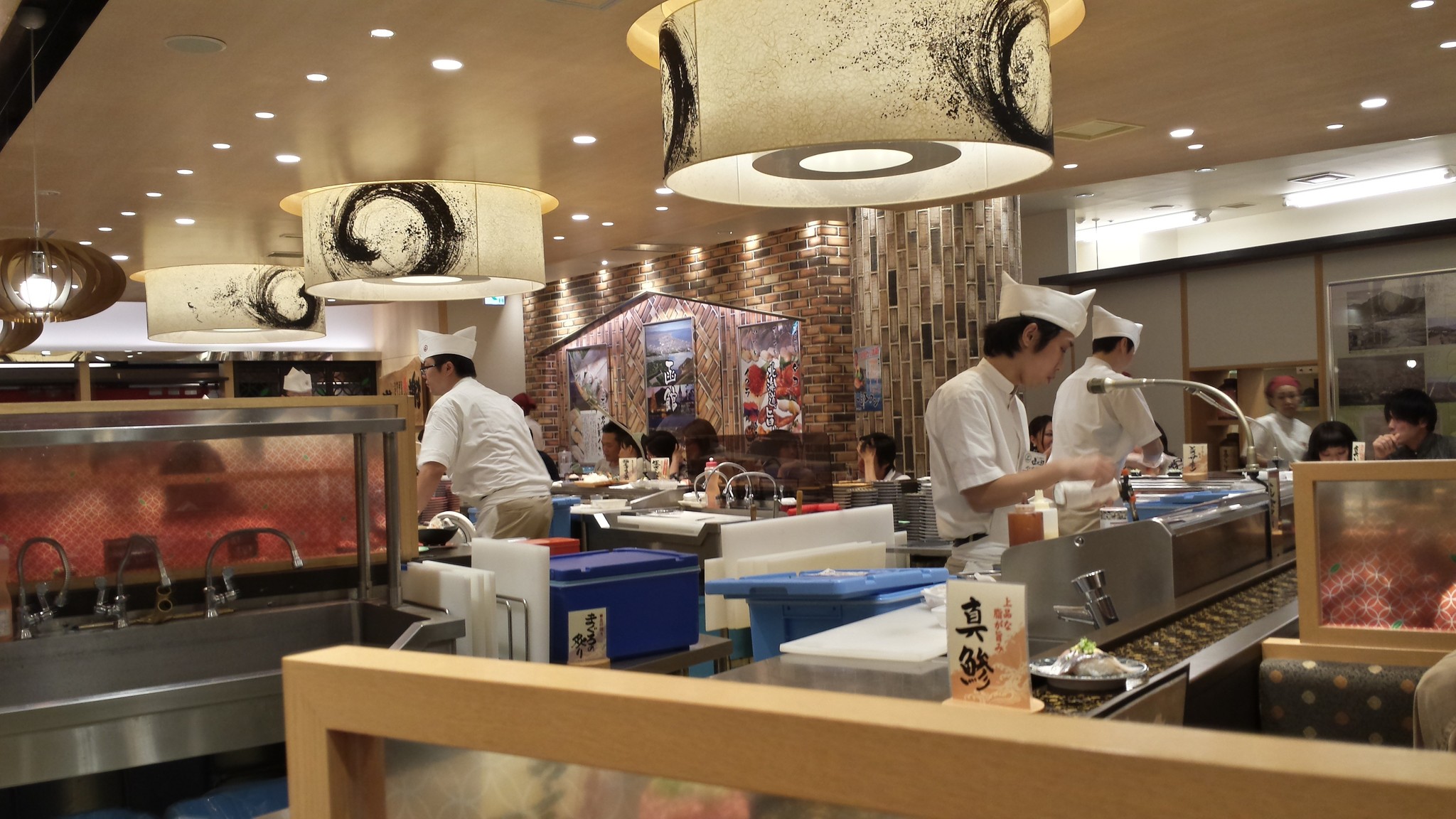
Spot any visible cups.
[590,494,603,500]
[572,495,582,505]
[571,463,583,474]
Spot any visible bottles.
[704,457,720,508]
[1215,378,1237,420]
[1007,492,1044,547]
[1031,490,1057,508]
[1219,433,1242,473]
[1034,502,1059,540]
[1122,469,1139,521]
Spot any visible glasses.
[420,362,441,375]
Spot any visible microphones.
[1179,383,1292,486]
[1087,376,1267,492]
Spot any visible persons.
[857,433,911,482]
[618,433,642,459]
[194,394,255,472]
[1028,305,1183,539]
[262,367,354,472]
[416,325,561,540]
[682,418,734,484]
[1372,388,1456,460]
[645,430,688,483]
[1240,375,1313,471]
[1302,421,1366,461]
[924,271,1117,576]
[756,429,822,487]
[37,440,284,580]
[593,421,628,481]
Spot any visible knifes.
[621,512,678,517]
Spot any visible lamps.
[1075,209,1212,242]
[0,6,127,355]
[625,1,1087,209]
[130,264,327,344]
[1279,165,1456,209]
[280,179,560,302]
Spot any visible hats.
[1266,375,1300,408]
[417,326,477,364]
[512,392,538,410]
[998,270,1097,337]
[1092,304,1143,355]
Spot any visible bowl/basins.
[590,498,627,509]
[418,525,460,546]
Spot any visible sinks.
[1,590,467,793]
[621,505,719,521]
[695,514,766,525]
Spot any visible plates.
[833,480,946,541]
[1029,655,1148,690]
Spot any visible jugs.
[558,450,572,478]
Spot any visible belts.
[953,533,988,547]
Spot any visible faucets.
[93,534,172,629]
[203,528,304,618]
[722,470,784,518]
[701,462,754,508]
[693,469,736,508]
[15,537,72,639]
[1053,568,1121,632]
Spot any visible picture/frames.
[642,317,698,463]
[566,343,612,465]
[737,319,805,460]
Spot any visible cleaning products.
[0,544,14,642]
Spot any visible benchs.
[1259,658,1431,747]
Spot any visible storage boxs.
[468,497,581,538]
[1123,491,1248,522]
[704,567,956,662]
[549,547,701,665]
